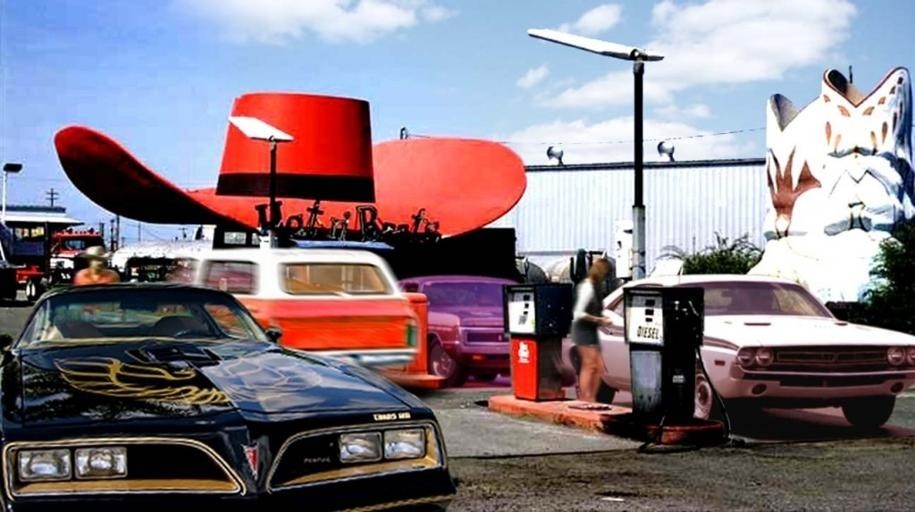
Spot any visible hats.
[54,91,528,237]
[76,246,114,259]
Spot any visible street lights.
[2,162,24,227]
[527,26,667,278]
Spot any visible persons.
[72,246,120,285]
[570,258,613,404]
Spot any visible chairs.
[43,320,103,343]
[152,316,214,341]
